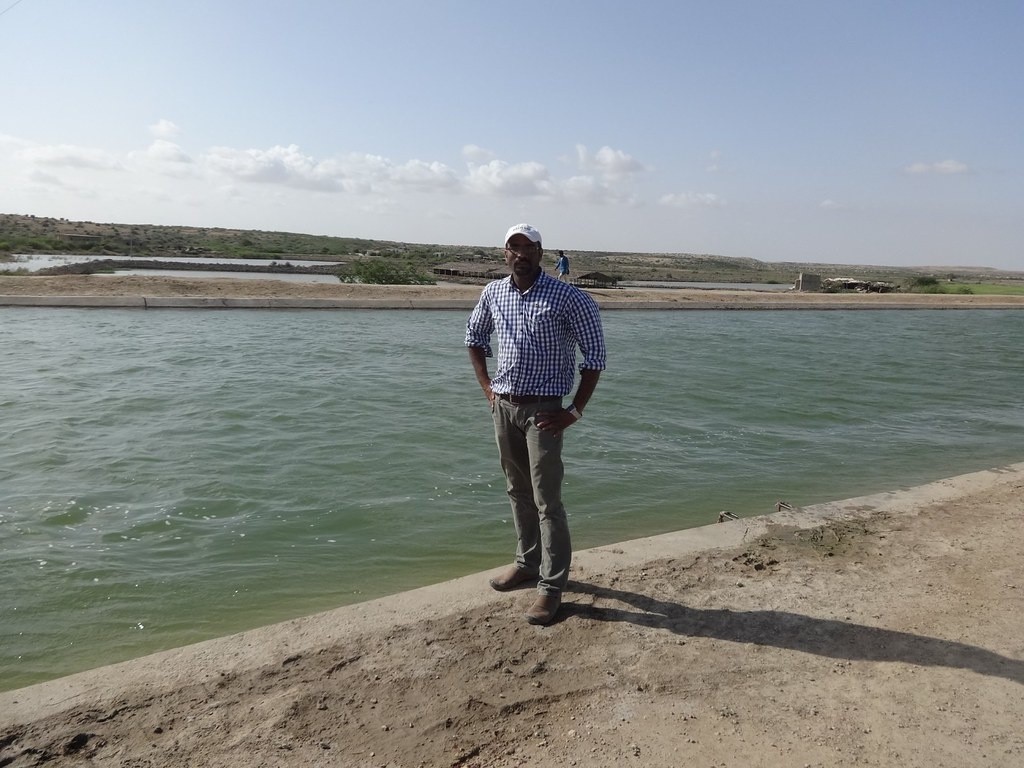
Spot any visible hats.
[504,223,541,248]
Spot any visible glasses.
[506,244,540,254]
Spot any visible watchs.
[567,404,583,420]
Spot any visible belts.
[494,393,559,404]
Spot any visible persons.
[464,223,608,626]
[555,250,569,284]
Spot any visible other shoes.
[524,595,560,626]
[489,566,533,591]
